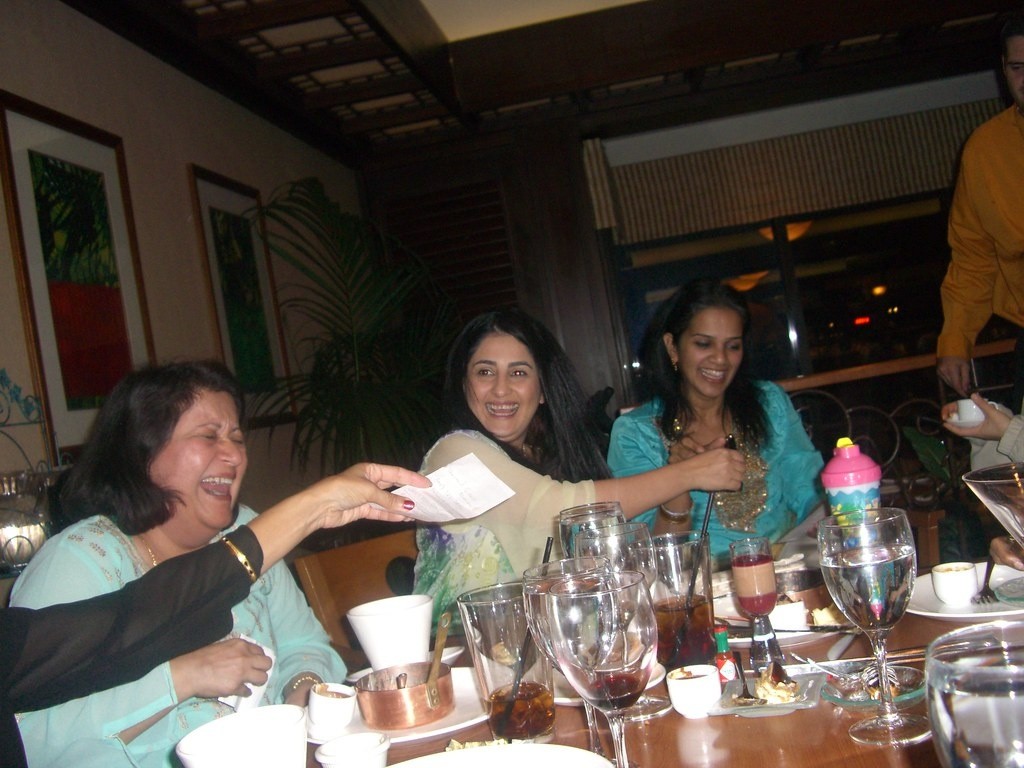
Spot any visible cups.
[932,562,978,604]
[962,460,1024,550]
[308,682,357,729]
[666,664,722,718]
[175,703,306,768]
[626,529,719,685]
[313,732,391,768]
[924,619,1024,768]
[957,398,998,422]
[347,593,434,672]
[458,579,557,744]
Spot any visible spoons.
[732,651,768,706]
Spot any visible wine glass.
[521,500,673,767]
[814,506,932,749]
[728,536,787,670]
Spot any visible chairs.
[294,528,418,655]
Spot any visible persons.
[605,280,831,574]
[940,393,1024,474]
[1,460,434,716]
[933,23,1024,404]
[0,356,348,768]
[410,311,747,638]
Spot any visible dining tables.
[306,585,979,768]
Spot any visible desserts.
[755,662,799,704]
[810,601,851,625]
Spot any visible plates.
[525,660,667,706]
[906,560,1024,622]
[706,671,826,718]
[712,593,842,651]
[305,665,492,746]
[947,416,983,427]
[380,743,613,767]
[346,645,465,682]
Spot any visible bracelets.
[658,500,695,526]
[136,530,157,569]
[222,536,258,585]
[292,675,321,689]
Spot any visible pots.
[354,612,456,730]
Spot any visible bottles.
[820,437,884,553]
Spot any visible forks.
[806,654,932,678]
[971,556,999,605]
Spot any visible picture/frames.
[0,89,159,472]
[187,162,297,429]
